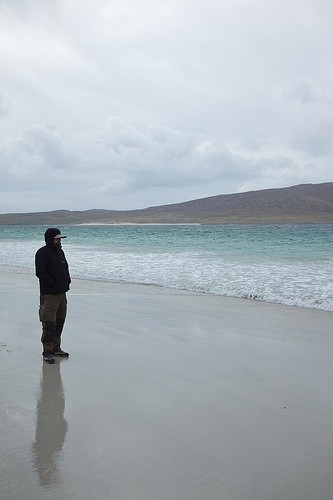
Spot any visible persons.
[29,362,69,490]
[33,228,72,364]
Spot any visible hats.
[53,233,69,240]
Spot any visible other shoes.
[42,353,56,365]
[53,349,69,357]
